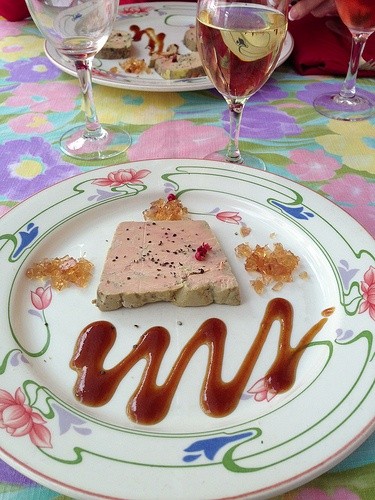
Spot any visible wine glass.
[313,0,375,119]
[198,1,290,172]
[26,0,133,161]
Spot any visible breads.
[91,30,134,60]
[148,50,214,78]
[96,220,242,309]
[183,25,200,51]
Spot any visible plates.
[42,2,294,92]
[1,159,375,499]
